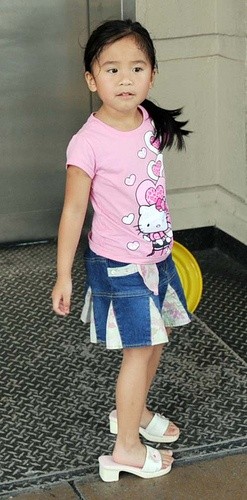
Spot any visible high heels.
[97,444,172,482]
[109,407,180,442]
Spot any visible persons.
[51,18,192,484]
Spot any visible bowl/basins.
[164,239,203,317]
